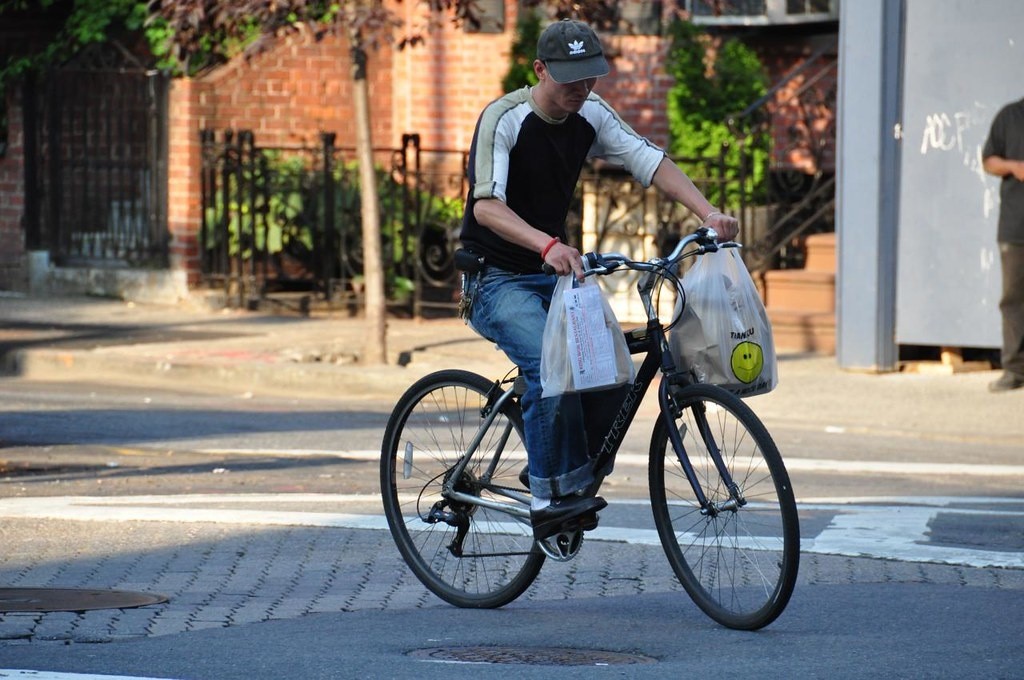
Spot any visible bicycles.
[379,225,800,631]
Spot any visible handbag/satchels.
[672,240,777,398]
[539,257,635,399]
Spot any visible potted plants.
[662,8,781,278]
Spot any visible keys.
[458,291,471,325]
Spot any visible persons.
[982,94,1024,391]
[458,17,739,562]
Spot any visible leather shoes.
[530,491,606,540]
[520,465,532,486]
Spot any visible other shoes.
[989,370,1024,392]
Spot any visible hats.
[536,18,609,85]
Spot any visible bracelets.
[703,212,721,223]
[541,237,560,260]
